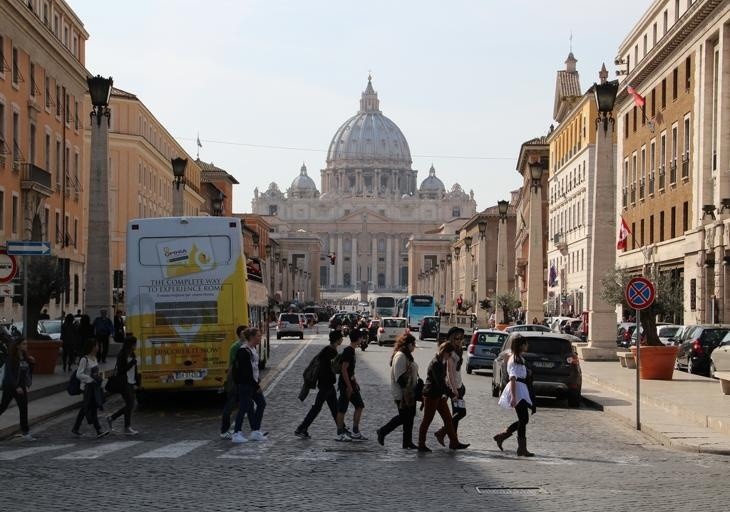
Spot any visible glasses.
[455,336,464,340]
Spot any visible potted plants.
[490,289,518,331]
[598,261,689,380]
[10,258,69,375]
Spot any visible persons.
[294,330,352,439]
[341,315,370,331]
[232,328,267,444]
[493,333,536,457]
[334,329,368,441]
[418,341,461,452]
[220,326,255,439]
[0,309,140,442]
[434,326,471,450]
[376,334,424,449]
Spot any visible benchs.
[712,371,730,395]
[616,352,636,370]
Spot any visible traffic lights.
[330,255,335,264]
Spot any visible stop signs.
[457,297,463,304]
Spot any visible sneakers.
[334,426,368,442]
[294,431,311,439]
[105,415,112,433]
[96,431,110,439]
[24,434,38,442]
[123,427,139,436]
[71,430,85,438]
[232,431,270,443]
[219,432,233,440]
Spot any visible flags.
[617,217,630,250]
[549,264,556,286]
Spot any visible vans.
[462,328,510,375]
[417,316,441,341]
[376,317,410,346]
[276,313,304,340]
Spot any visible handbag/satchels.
[412,379,425,411]
[105,376,123,393]
[67,369,81,395]
[0,363,6,390]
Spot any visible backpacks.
[330,349,354,374]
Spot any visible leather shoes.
[403,442,419,449]
[453,442,470,449]
[419,446,432,453]
[377,429,384,445]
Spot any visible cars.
[709,331,730,378]
[300,305,379,351]
[489,330,582,408]
[544,315,589,342]
[615,321,730,374]
[9,319,75,352]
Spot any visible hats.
[83,338,96,353]
[350,329,365,341]
[446,326,464,341]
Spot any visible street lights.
[264,244,273,298]
[274,251,312,303]
[495,199,510,329]
[525,160,547,326]
[252,231,261,257]
[210,188,223,217]
[172,157,187,217]
[576,80,631,361]
[417,219,488,328]
[85,74,123,358]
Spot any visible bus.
[123,215,280,412]
[369,296,397,321]
[396,296,405,317]
[401,294,436,330]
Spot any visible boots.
[435,426,448,447]
[493,431,513,451]
[517,437,534,457]
